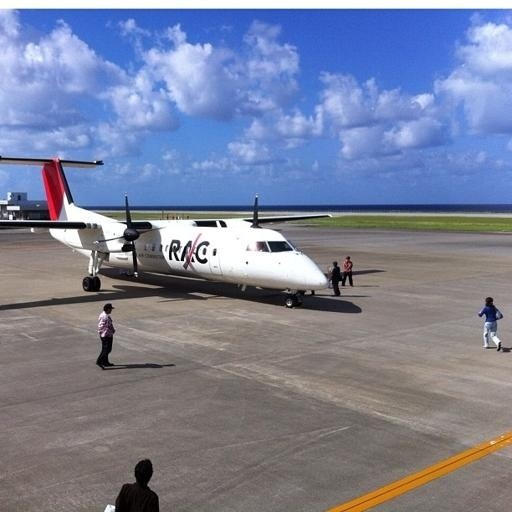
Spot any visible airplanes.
[0,156,330,307]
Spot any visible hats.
[104,304,115,311]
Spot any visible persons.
[477,296,504,352]
[328,261,342,297]
[114,458,159,512]
[342,255,354,287]
[94,303,116,370]
